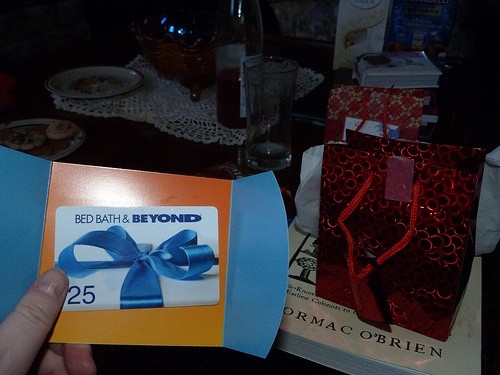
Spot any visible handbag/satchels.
[314,137,488,342]
[289,140,341,234]
[319,84,422,145]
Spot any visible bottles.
[216,0,263,127]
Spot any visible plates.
[46,65,143,101]
[2,118,83,160]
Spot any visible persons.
[1,268,97,375]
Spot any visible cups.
[241,55,299,171]
